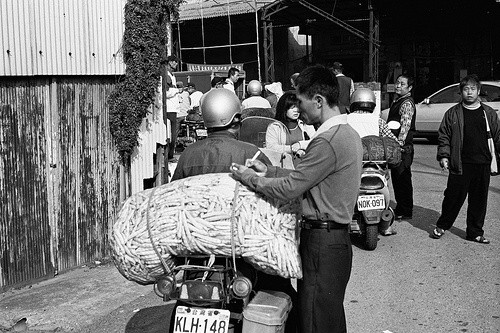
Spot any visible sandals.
[466,236,490,243]
[432,226,446,237]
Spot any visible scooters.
[174,116,208,154]
[153,254,294,333]
[351,120,401,251]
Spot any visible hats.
[187,83,194,88]
[330,62,344,71]
[264,82,282,95]
[212,77,228,85]
[167,55,181,61]
[176,82,185,88]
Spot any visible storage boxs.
[242,289,292,333]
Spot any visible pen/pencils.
[246,150,261,168]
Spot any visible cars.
[381,80,500,144]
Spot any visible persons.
[230,64,363,333]
[432,75,500,244]
[170,88,272,183]
[165,56,180,162]
[387,74,416,220]
[266,91,311,160]
[175,67,301,130]
[330,62,355,114]
[345,87,402,235]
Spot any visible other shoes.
[393,208,412,220]
[384,227,398,235]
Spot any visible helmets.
[200,88,242,127]
[350,87,376,104]
[247,80,263,95]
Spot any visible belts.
[298,219,349,229]
